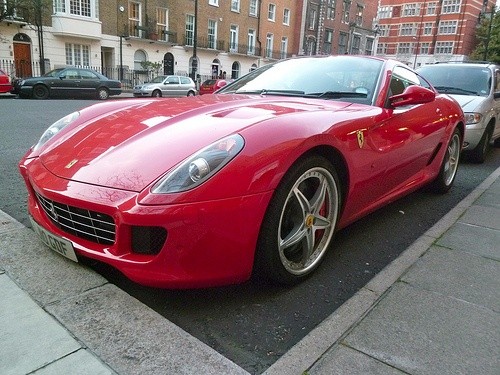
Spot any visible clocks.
[120,6,125,12]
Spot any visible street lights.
[119,35,130,82]
[480,0,500,63]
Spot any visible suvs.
[418,61,500,165]
[135,76,198,97]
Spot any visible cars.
[0,70,13,94]
[13,68,123,100]
[200,79,227,95]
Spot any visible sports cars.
[17,54,467,290]
